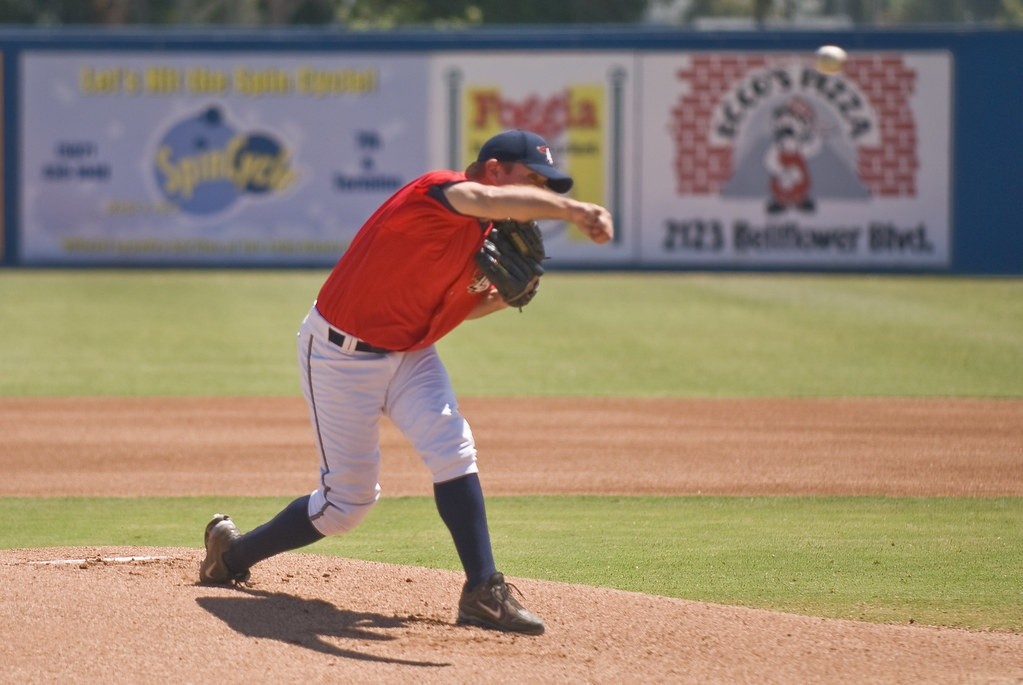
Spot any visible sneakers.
[456,571,545,636]
[199,515,252,586]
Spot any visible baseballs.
[814,44,849,76]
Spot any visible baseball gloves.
[474,217,552,314]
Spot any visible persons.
[200,129,614,636]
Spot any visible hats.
[477,128,573,194]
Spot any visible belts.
[329,329,389,353]
